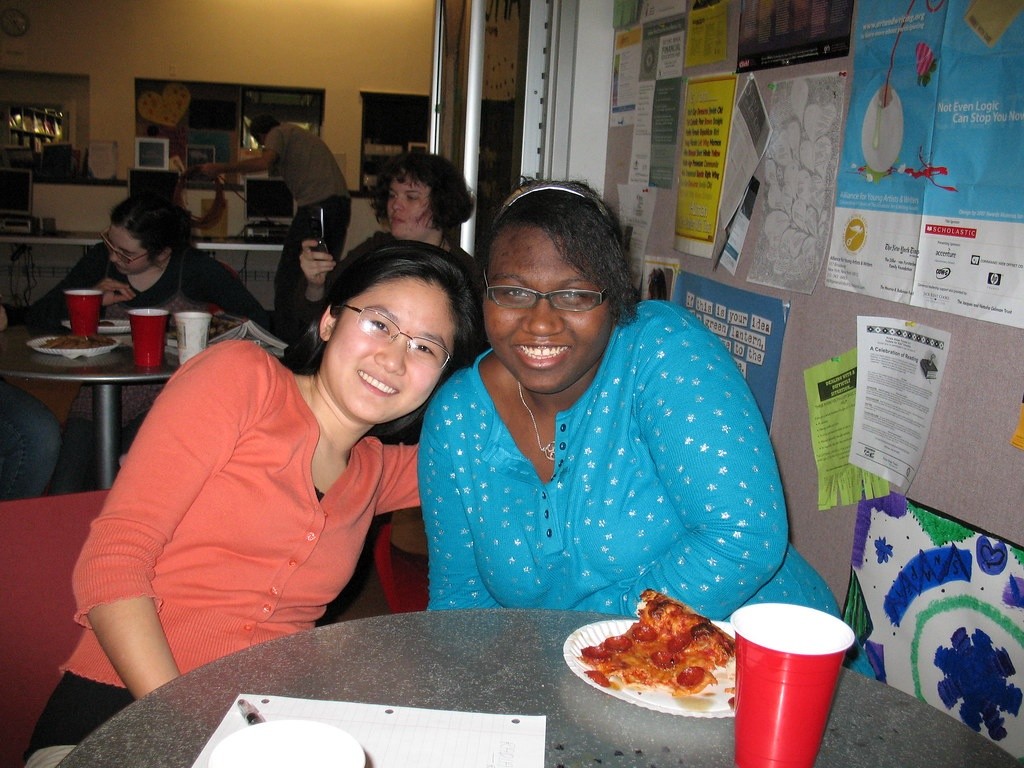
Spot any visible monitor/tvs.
[246,177,295,224]
[0,167,33,215]
[127,167,176,206]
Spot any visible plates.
[211,721,366,768]
[564,618,751,721]
[61,318,133,334]
[26,335,121,358]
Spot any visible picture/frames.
[134,138,170,171]
[186,144,215,169]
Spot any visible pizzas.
[580,587,737,698]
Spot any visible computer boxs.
[0,215,38,236]
[244,222,289,244]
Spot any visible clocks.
[0,6,27,35]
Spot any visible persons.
[275,152,491,527]
[24,240,489,768]
[250,114,353,338]
[0,193,270,497]
[418,180,875,683]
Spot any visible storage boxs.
[31,180,129,233]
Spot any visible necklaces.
[517,381,554,461]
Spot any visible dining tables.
[1,315,275,490]
[53,608,1024,768]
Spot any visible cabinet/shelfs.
[0,102,65,153]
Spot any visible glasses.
[345,304,452,370]
[481,269,606,312]
[100,227,151,265]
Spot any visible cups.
[174,311,213,367]
[128,308,170,367]
[732,601,858,768]
[64,289,103,338]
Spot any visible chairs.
[0,490,109,768]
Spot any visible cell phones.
[308,205,330,255]
